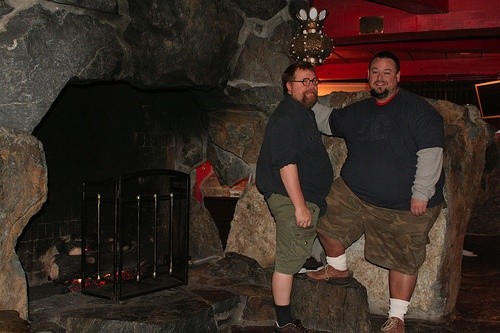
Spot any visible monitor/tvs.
[475,80,500,119]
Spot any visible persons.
[306,50,448,333]
[255,61,334,333]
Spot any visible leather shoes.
[380,316,405,333]
[306,264,351,284]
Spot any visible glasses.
[288,77,320,86]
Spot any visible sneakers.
[273,319,313,333]
[298,256,325,274]
